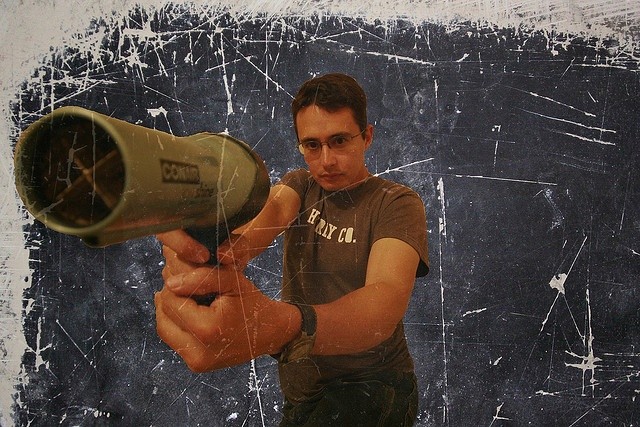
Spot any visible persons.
[154,73,429,426]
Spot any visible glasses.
[298,129,365,156]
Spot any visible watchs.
[269,300,316,365]
